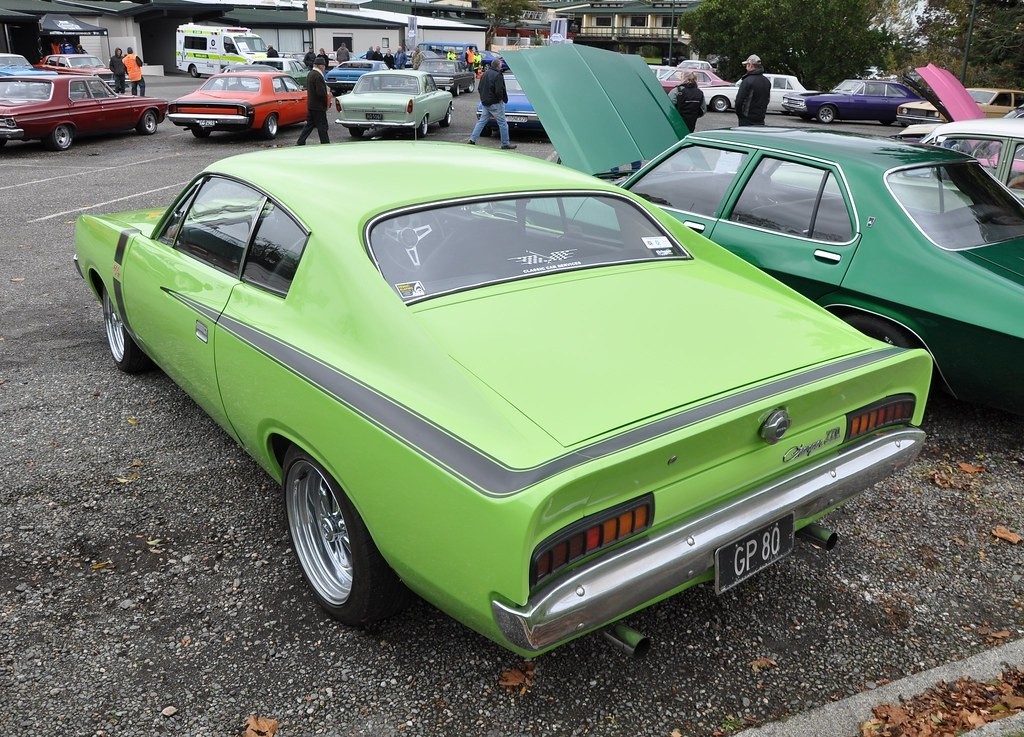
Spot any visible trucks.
[176,22,269,78]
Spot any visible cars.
[700,73,819,114]
[355,41,510,73]
[769,63,1024,214]
[212,64,298,91]
[324,60,391,96]
[334,70,454,138]
[0,53,59,93]
[253,51,358,82]
[32,54,115,92]
[165,71,334,140]
[650,60,734,94]
[465,41,1024,414]
[477,75,547,137]
[408,59,475,97]
[0,76,168,151]
[74,141,934,661]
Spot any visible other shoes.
[501,145,517,149]
[467,140,475,145]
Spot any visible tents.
[39,13,111,60]
[0,8,37,54]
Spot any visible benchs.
[747,197,847,238]
[71,92,85,99]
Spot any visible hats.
[313,58,326,64]
[742,54,761,65]
[309,46,312,49]
[127,47,132,52]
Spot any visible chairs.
[228,83,246,90]
[859,87,870,94]
[416,216,524,277]
[872,87,881,94]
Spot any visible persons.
[316,49,329,76]
[735,54,771,127]
[304,47,316,71]
[447,48,457,67]
[384,49,394,70]
[467,59,517,149]
[110,48,128,94]
[373,46,384,61]
[366,46,374,60]
[51,38,88,55]
[465,46,474,73]
[337,43,350,64]
[296,57,330,145]
[474,50,482,74]
[411,46,422,71]
[668,69,707,132]
[267,45,279,67]
[394,46,407,69]
[123,47,145,96]
